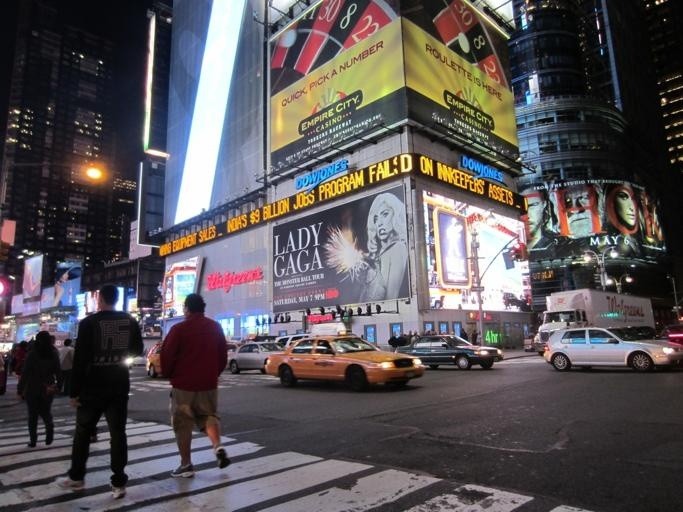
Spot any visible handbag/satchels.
[40,380,59,397]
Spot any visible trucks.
[532,290,659,355]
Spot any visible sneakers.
[215,447,232,470]
[54,475,85,491]
[111,482,126,499]
[170,464,195,478]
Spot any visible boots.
[28,421,37,447]
[45,423,54,445]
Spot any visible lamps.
[252,300,383,327]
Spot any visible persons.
[161,294,231,479]
[522,178,667,264]
[57,285,142,500]
[356,194,407,304]
[1,331,81,449]
[390,327,478,350]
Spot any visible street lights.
[0,159,104,186]
[580,244,615,291]
[666,275,680,322]
[466,227,484,347]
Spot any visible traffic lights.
[514,244,525,261]
